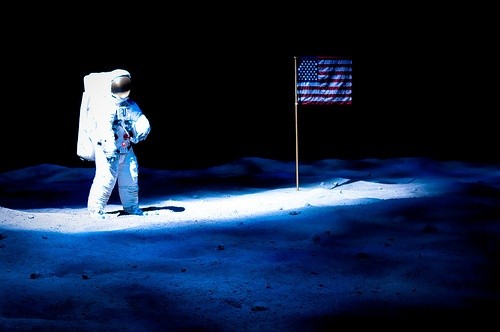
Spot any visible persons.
[87,67,152,217]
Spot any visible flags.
[296,57,353,105]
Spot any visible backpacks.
[76,72,108,162]
